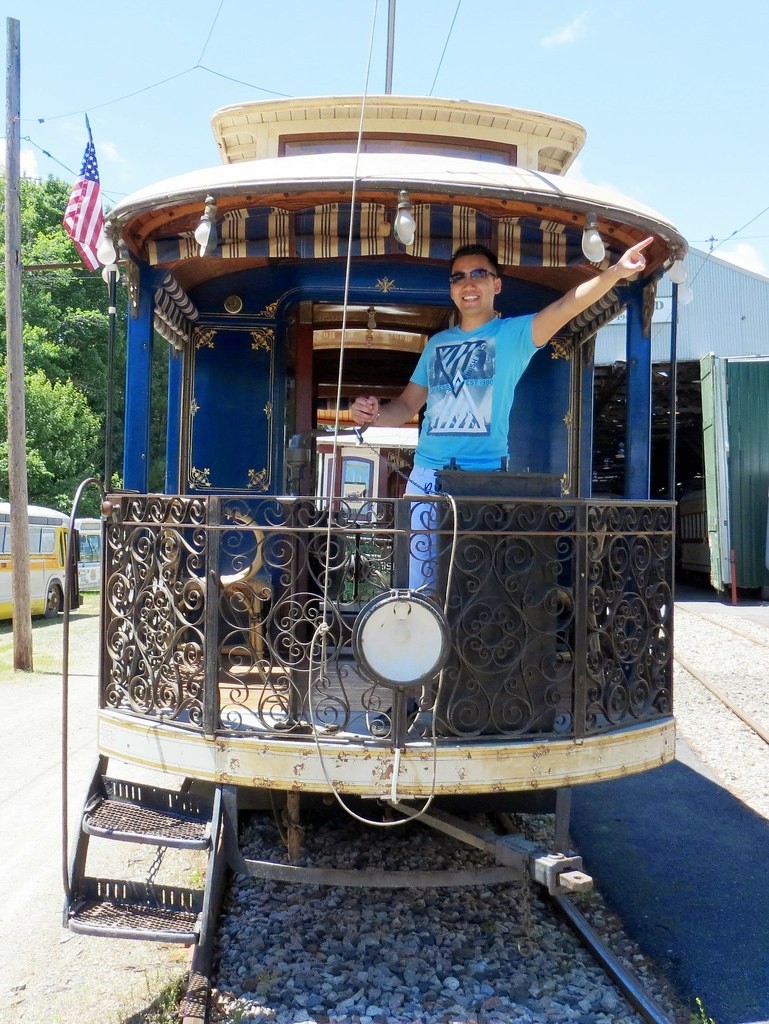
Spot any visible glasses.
[449,268,496,285]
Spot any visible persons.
[347,238,655,735]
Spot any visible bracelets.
[371,407,381,425]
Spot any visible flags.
[64,138,111,273]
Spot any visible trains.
[57,93,688,943]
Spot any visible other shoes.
[370,699,419,734]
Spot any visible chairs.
[111,486,273,684]
[412,452,609,722]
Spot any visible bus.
[74,518,101,592]
[0,502,80,625]
